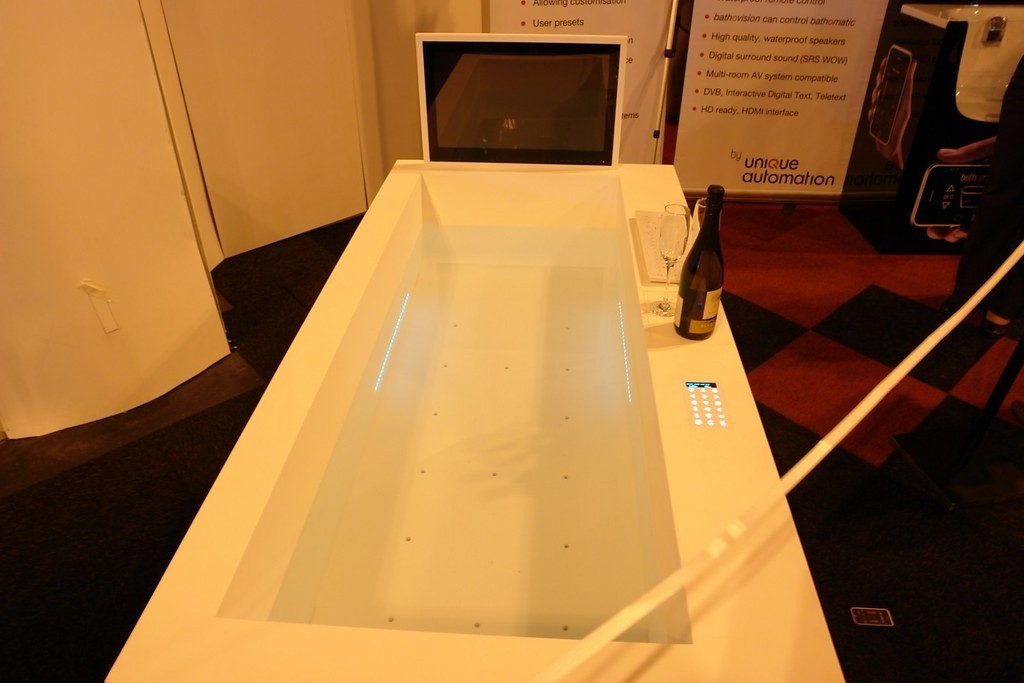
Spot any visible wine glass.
[688,197,722,252]
[651,204,690,317]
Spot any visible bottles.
[673,184,726,341]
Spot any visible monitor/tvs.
[414,31,628,173]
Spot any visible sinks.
[97,157,851,683]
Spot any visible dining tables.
[104,155,846,683]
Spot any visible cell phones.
[868,44,912,147]
[910,162,990,228]
